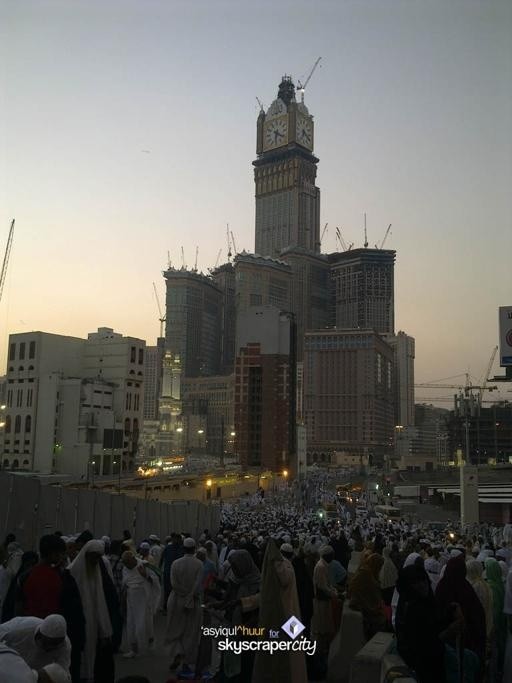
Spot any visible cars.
[355,505,368,516]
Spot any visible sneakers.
[121,649,137,658]
[169,654,193,672]
[145,637,158,650]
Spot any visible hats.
[38,612,68,639]
[41,661,70,682]
[0,508,512,575]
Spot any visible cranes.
[412,345,508,405]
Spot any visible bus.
[375,506,400,522]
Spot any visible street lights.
[395,425,404,456]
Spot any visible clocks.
[292,109,314,151]
[262,113,288,152]
